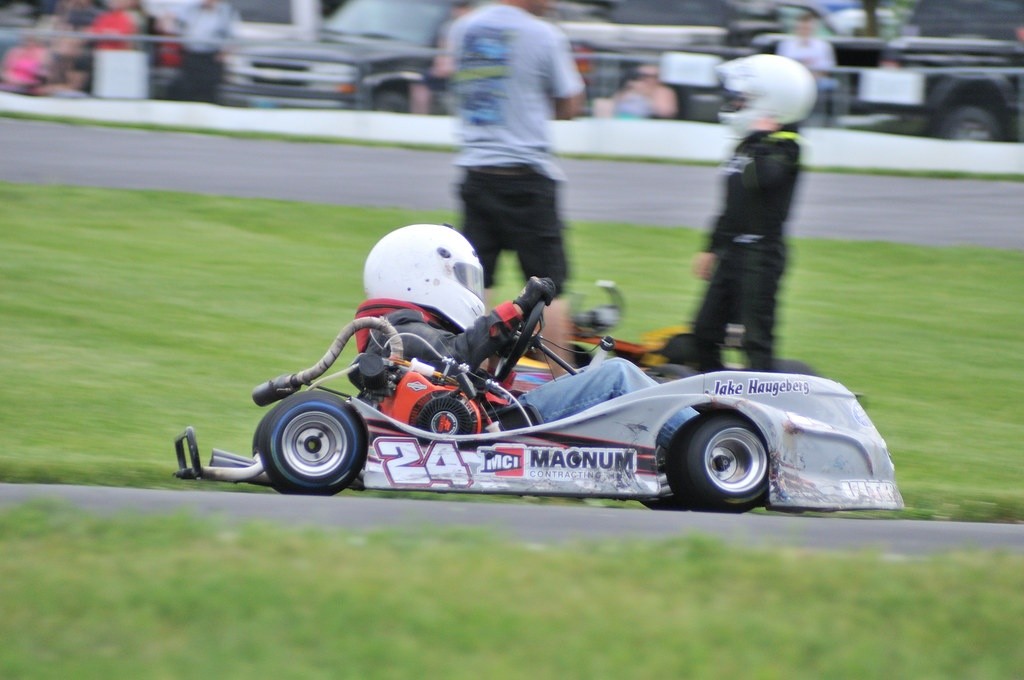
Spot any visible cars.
[2,0,852,144]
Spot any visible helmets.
[714,54,818,139]
[363,223,485,330]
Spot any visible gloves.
[513,276,556,314]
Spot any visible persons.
[689,53,817,371]
[0,0,240,102]
[778,10,836,115]
[593,63,678,119]
[447,0,585,373]
[355,224,699,448]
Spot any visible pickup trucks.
[658,0,1024,145]
